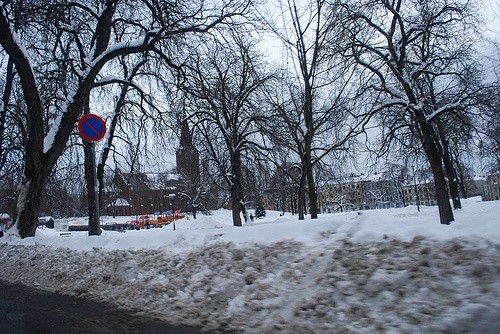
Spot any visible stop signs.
[78,113,106,142]
